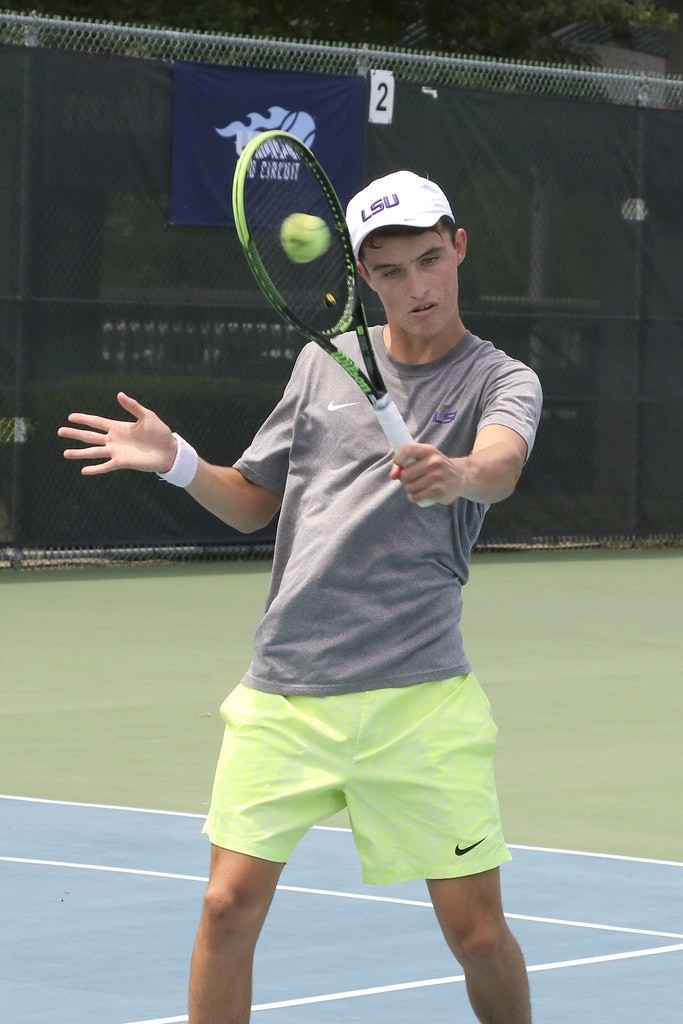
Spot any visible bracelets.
[156,432,198,488]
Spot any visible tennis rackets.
[232,128,432,511]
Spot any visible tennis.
[279,213,333,264]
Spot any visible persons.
[59,167,532,1021]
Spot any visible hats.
[345,171,456,262]
